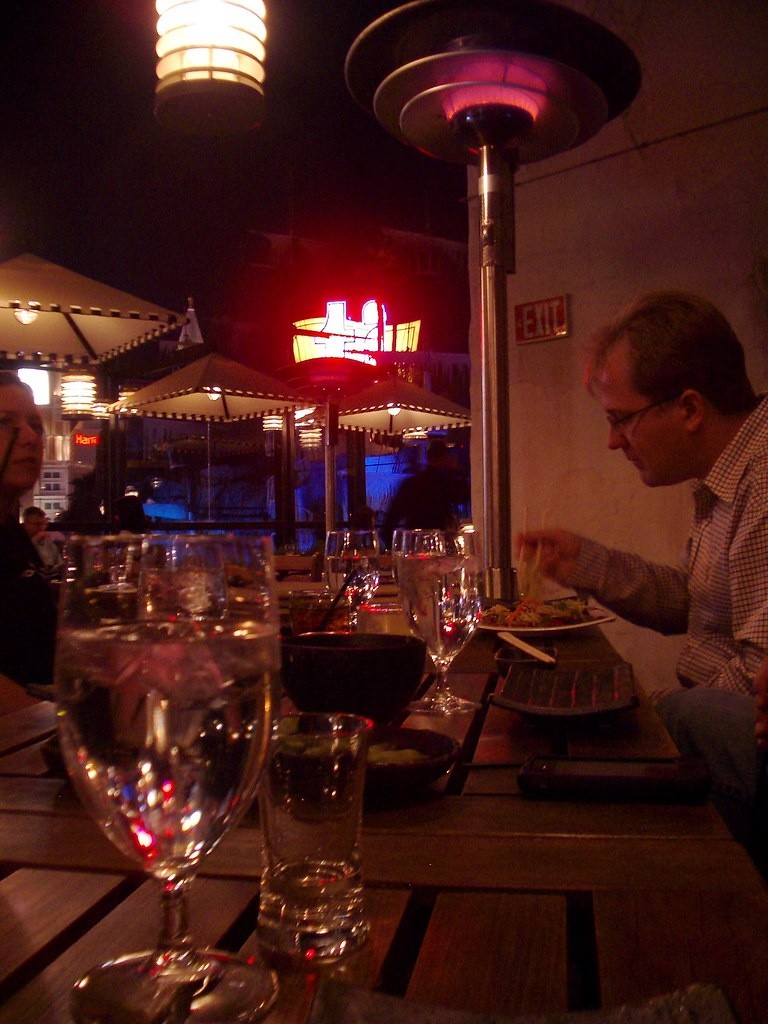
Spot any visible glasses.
[606,386,689,438]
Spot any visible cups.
[392,527,444,637]
[485,568,520,606]
[288,589,352,634]
[256,713,370,966]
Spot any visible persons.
[0,383,58,682]
[385,442,459,548]
[22,506,64,577]
[518,290,767,841]
[110,495,148,533]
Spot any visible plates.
[368,726,460,792]
[477,616,617,642]
[485,660,641,725]
[493,644,560,678]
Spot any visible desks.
[0,566,768,1024]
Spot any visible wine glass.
[397,551,484,714]
[326,531,379,630]
[55,534,279,1024]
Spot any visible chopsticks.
[516,505,549,574]
[496,631,557,664]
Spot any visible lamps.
[298,427,323,451]
[344,0,643,615]
[58,370,142,421]
[0,350,90,364]
[261,415,284,430]
[386,402,401,417]
[206,386,222,400]
[400,429,427,446]
[152,0,268,143]
[9,309,39,325]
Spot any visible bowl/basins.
[280,632,426,732]
